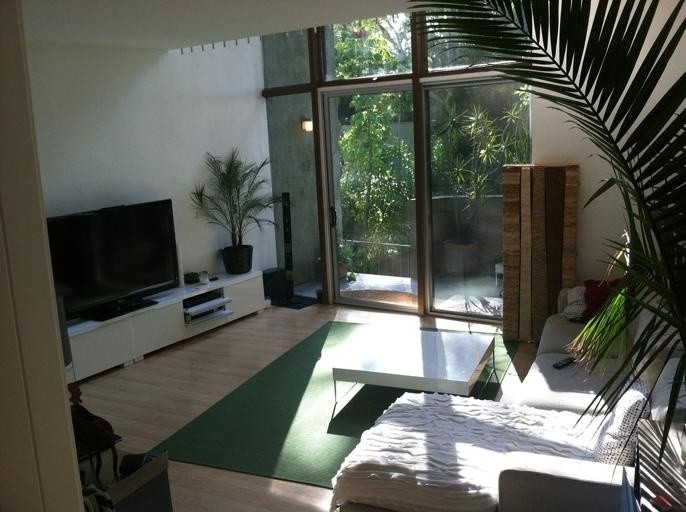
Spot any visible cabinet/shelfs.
[64,269,266,385]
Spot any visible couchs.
[328,288,686,512]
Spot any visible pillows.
[583,278,622,323]
[563,286,587,319]
[593,389,650,466]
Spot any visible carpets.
[148,321,520,490]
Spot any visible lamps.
[301,118,313,132]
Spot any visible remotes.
[553,356,577,369]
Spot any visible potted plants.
[430,85,532,277]
[190,146,295,274]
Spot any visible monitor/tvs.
[45,198,180,322]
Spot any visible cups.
[199,271,209,284]
